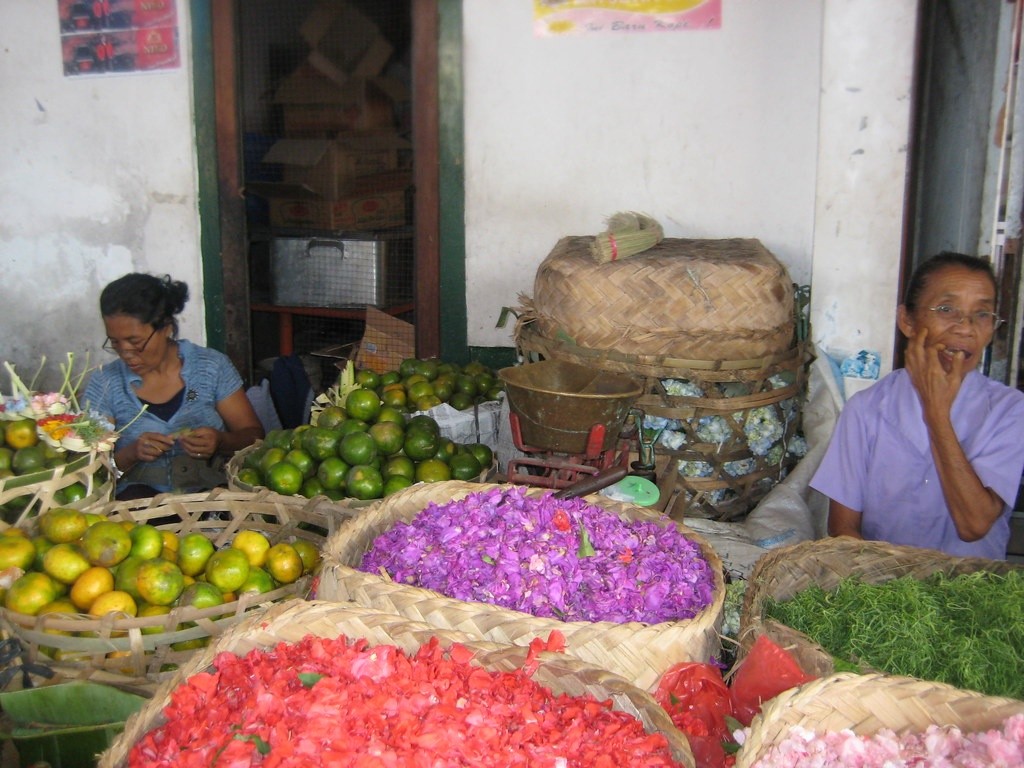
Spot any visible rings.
[198,453,200,458]
[145,440,148,446]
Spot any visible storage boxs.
[268,3,415,308]
[351,305,415,375]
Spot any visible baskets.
[513,233,813,519]
[2,418,1022,767]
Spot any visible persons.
[80,273,267,526]
[807,252,1024,561]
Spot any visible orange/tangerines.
[240,358,504,538]
[0,417,319,674]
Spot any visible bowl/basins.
[498,359,644,454]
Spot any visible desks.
[250,296,412,359]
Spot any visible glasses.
[102,320,163,355]
[907,304,1006,331]
